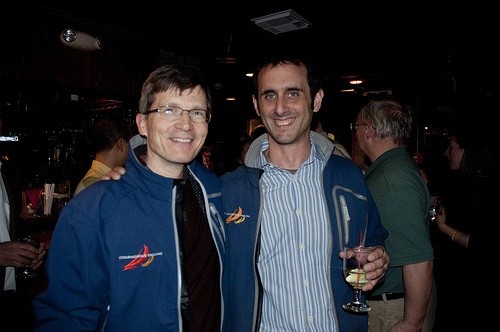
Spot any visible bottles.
[428,192,440,223]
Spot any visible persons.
[73,119,130,197]
[99,51,389,332]
[430,127,500,332]
[0,161,46,332]
[356,102,437,332]
[33,64,389,332]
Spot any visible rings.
[375,245,385,256]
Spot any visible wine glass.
[17,237,37,278]
[343,247,372,312]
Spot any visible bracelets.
[451,230,457,241]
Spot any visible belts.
[365,292,405,302]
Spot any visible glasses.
[144,106,212,123]
[350,123,377,131]
